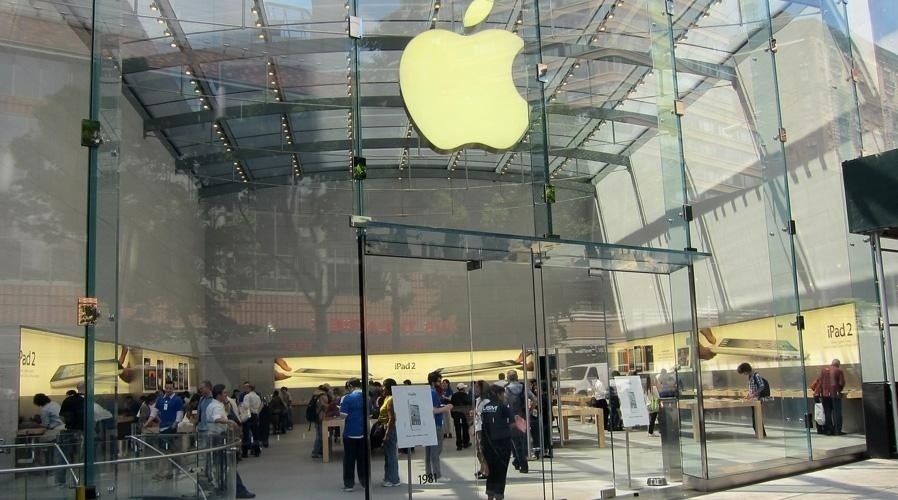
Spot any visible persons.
[192,381,255,498]
[425,371,452,482]
[481,384,516,499]
[811,359,846,435]
[311,383,343,457]
[679,348,689,366]
[441,379,454,438]
[336,376,401,492]
[77,381,113,422]
[143,381,184,481]
[403,379,411,385]
[239,381,263,457]
[137,390,202,469]
[123,395,140,423]
[35,393,64,477]
[587,368,684,437]
[268,390,287,435]
[257,391,269,448]
[451,382,472,451]
[281,387,294,430]
[55,390,85,488]
[504,369,537,474]
[468,380,490,479]
[528,378,551,459]
[737,363,767,437]
[493,373,508,387]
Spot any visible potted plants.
[142,356,189,391]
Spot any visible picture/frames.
[18,412,139,472]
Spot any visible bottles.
[457,382,468,389]
[321,383,330,390]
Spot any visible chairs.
[434,476,451,483]
[475,471,488,479]
[383,480,400,487]
[239,490,255,497]
[464,442,472,448]
[311,452,322,458]
[341,485,353,492]
[649,433,658,437]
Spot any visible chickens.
[617,344,653,373]
[433,360,523,377]
[50,359,123,388]
[284,367,383,380]
[706,336,810,360]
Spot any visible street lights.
[814,402,826,425]
[240,406,252,422]
[370,420,385,448]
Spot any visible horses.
[627,389,639,413]
[144,358,188,390]
[408,398,422,431]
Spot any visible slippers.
[647,392,659,411]
[753,372,770,397]
[306,394,325,422]
[509,388,525,416]
